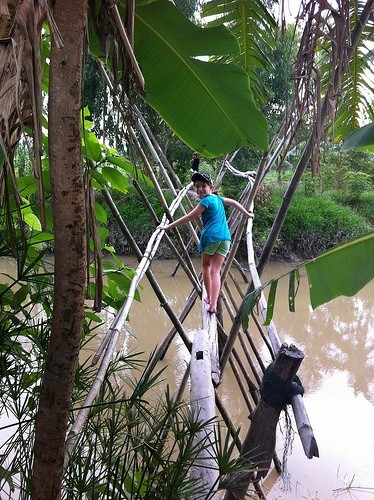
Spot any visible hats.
[190,172,213,185]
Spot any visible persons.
[156,172,254,313]
[191,154,200,178]
